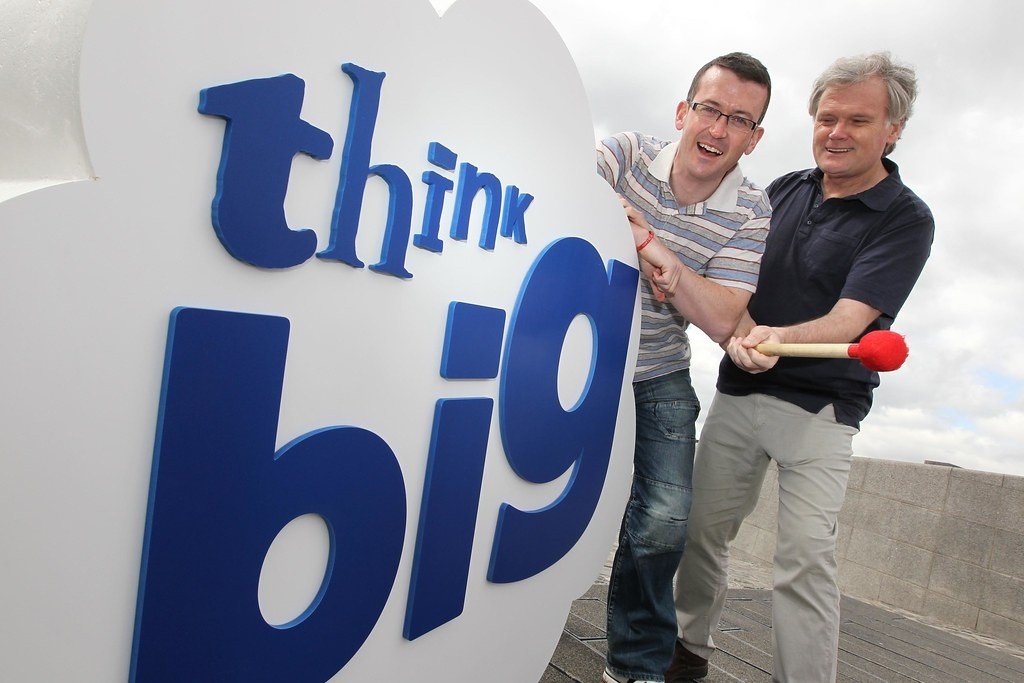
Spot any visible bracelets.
[636,232,655,252]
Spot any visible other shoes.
[665,641,709,683]
[602,668,664,683]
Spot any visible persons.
[667,53,937,683]
[596,50,773,683]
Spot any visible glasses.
[688,102,759,132]
